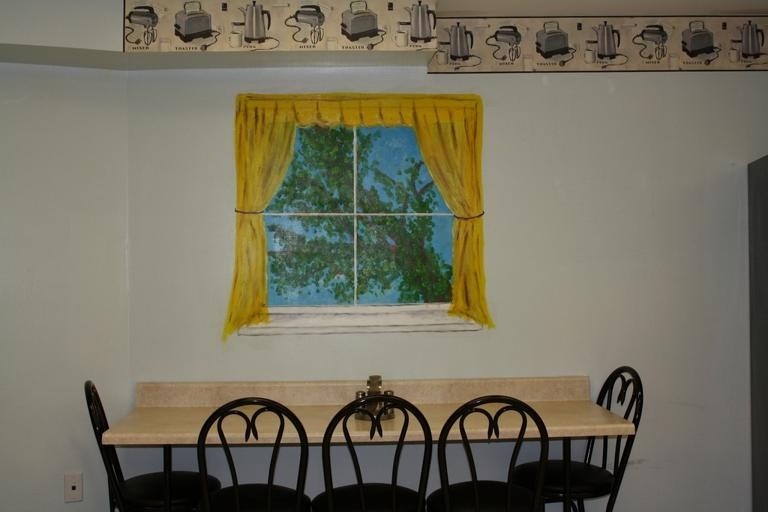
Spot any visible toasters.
[339,1,379,42]
[172,2,212,42]
[680,20,715,58]
[534,20,570,60]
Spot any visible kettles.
[734,20,766,59]
[402,0,438,44]
[442,20,476,61]
[238,2,272,45]
[590,20,621,61]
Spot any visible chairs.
[195,391,307,512]
[506,363,647,511]
[424,391,552,512]
[311,392,433,511]
[80,377,221,511]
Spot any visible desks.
[100,371,641,510]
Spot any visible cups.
[725,47,742,64]
[435,49,448,66]
[579,50,596,64]
[225,32,243,49]
[391,32,409,48]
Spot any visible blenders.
[291,4,325,44]
[639,24,668,59]
[123,5,158,45]
[492,25,521,61]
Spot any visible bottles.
[355,391,366,417]
[367,375,383,417]
[383,390,394,416]
